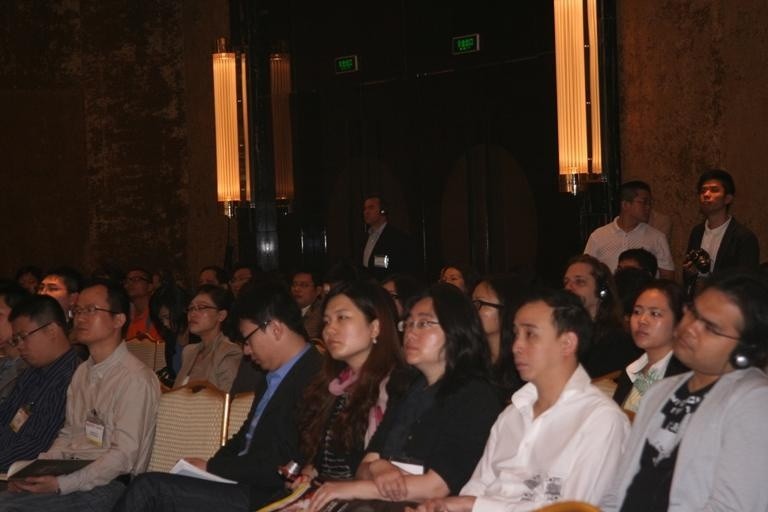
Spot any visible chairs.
[153,381,231,486]
[126,332,155,372]
[227,389,256,445]
[153,339,165,371]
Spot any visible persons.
[683,169,759,290]
[356,194,402,285]
[0,247,766,512]
[583,181,675,278]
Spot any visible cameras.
[685,248,711,273]
[274,459,301,482]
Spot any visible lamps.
[212,36,251,218]
[553,0,602,196]
[271,52,295,216]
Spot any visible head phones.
[730,341,768,369]
[598,286,609,299]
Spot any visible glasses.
[8,320,62,347]
[124,276,149,285]
[67,305,119,319]
[397,319,440,332]
[473,300,503,311]
[184,305,218,314]
